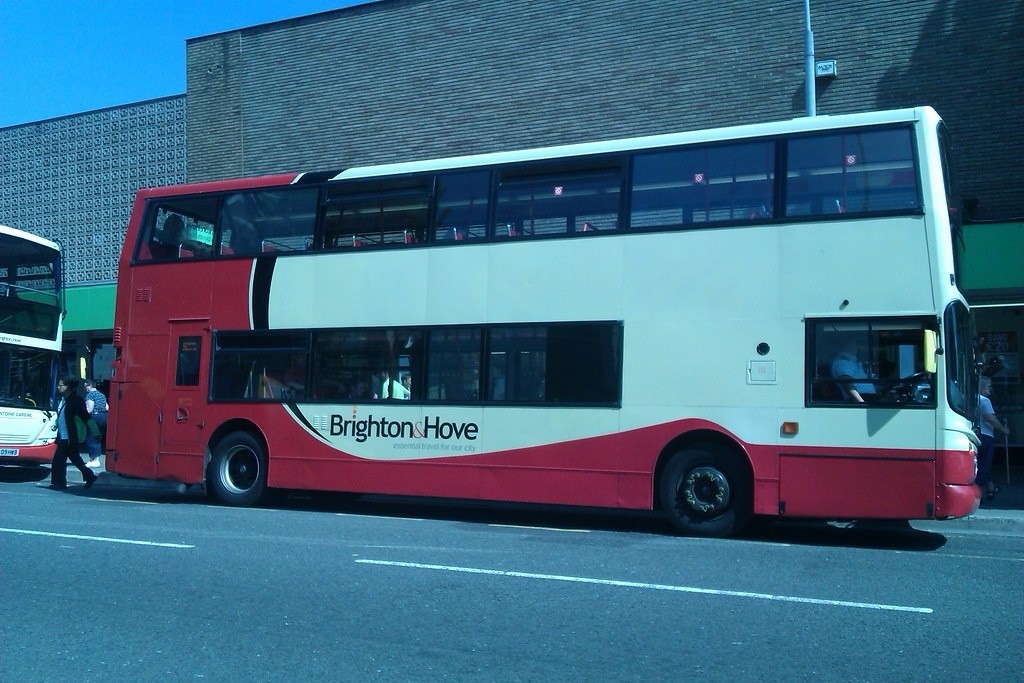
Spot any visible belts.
[90,411,108,414]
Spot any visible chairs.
[152,221,587,257]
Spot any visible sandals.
[987,486,1001,499]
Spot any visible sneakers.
[85,457,100,468]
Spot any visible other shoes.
[50,485,66,489]
[86,475,97,488]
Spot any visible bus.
[106,106,993,537]
[0,225,66,468]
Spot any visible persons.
[84,379,109,468]
[978,375,1010,499]
[492,363,505,401]
[401,374,411,399]
[831,336,876,402]
[343,375,367,398]
[49,376,101,489]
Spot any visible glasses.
[58,384,66,387]
[85,385,90,387]
